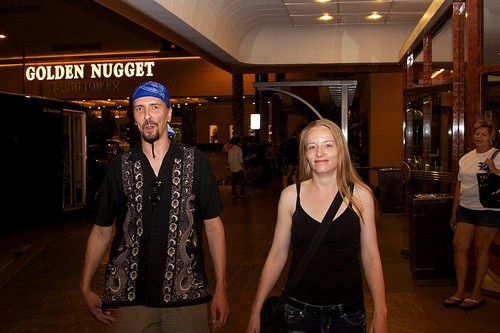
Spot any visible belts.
[287,298,362,317]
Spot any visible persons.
[246,119,387,333]
[223,134,299,200]
[79,82,229,333]
[443,121,500,310]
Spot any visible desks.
[408,193,455,280]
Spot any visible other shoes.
[240,195,247,198]
[232,195,237,199]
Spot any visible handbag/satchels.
[476,150,500,209]
[260,296,287,333]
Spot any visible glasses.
[149,177,162,208]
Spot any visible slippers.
[444,297,463,307]
[460,298,485,310]
